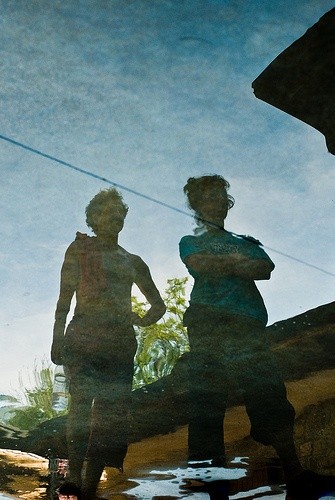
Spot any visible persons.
[49,189,169,499]
[175,173,330,498]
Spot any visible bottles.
[52,355,71,411]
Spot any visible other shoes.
[59,480,80,497]
